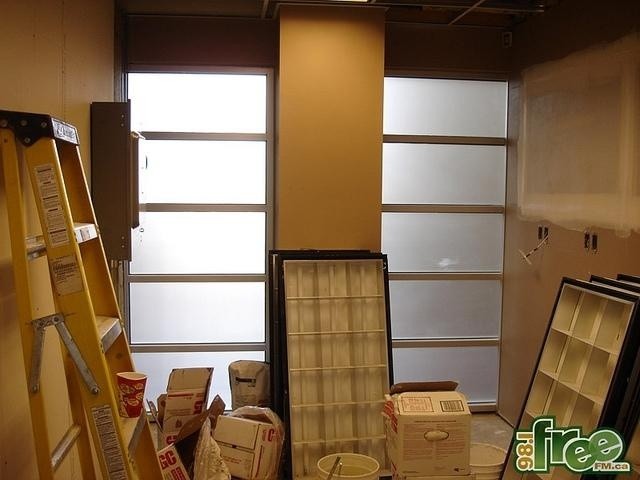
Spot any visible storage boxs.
[154,366,215,452]
[383,380,471,479]
[211,414,281,480]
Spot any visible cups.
[117,371,147,418]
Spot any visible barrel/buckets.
[469,442,507,480]
[317,453,380,479]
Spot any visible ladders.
[0,110,164,480]
